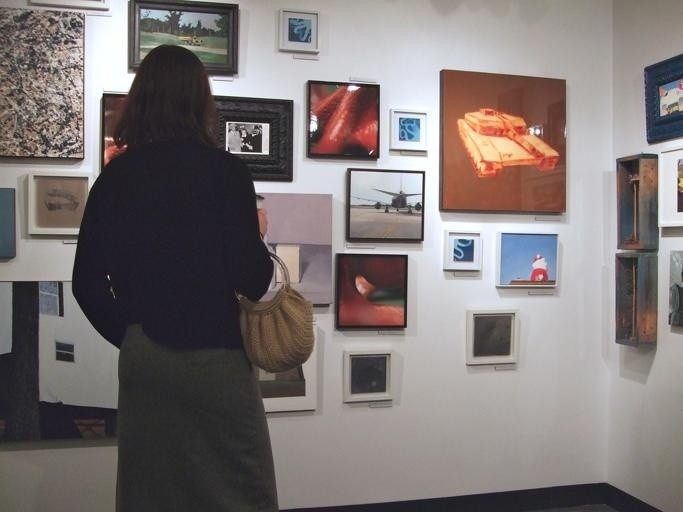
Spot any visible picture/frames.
[99,90,128,172]
[388,109,428,152]
[642,52,681,143]
[304,79,381,160]
[656,146,683,227]
[465,308,519,366]
[441,230,483,272]
[125,0,241,73]
[276,8,320,55]
[23,171,92,237]
[211,94,293,181]
[335,252,409,331]
[493,230,559,290]
[342,349,392,402]
[344,168,425,243]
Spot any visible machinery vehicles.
[457,107,558,180]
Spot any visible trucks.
[177,34,203,47]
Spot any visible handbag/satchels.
[239,284,315,374]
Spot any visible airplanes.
[351,173,421,214]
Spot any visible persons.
[226,123,262,154]
[67,43,278,512]
